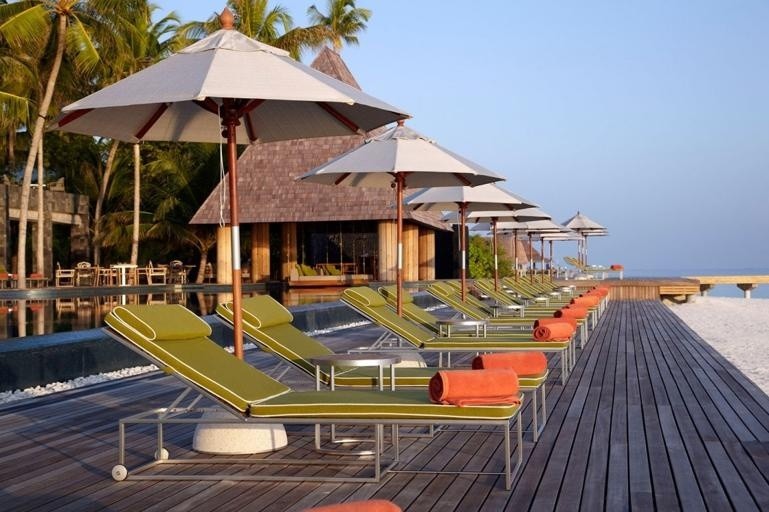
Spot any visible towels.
[533,318,577,331]
[554,310,586,319]
[299,499,402,512]
[591,286,608,295]
[584,290,602,298]
[428,367,521,408]
[30,274,43,278]
[472,351,548,376]
[570,298,594,307]
[533,323,573,341]
[611,264,623,269]
[579,294,599,304]
[0,273,8,278]
[562,305,589,310]
[11,274,17,278]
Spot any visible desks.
[75,267,95,286]
[490,304,524,331]
[561,285,576,295]
[111,264,138,287]
[522,297,549,307]
[175,264,196,284]
[309,353,401,463]
[434,319,488,368]
[548,292,561,302]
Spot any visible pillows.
[295,264,304,276]
[301,264,317,276]
[322,264,330,275]
[326,264,341,275]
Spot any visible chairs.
[446,280,589,342]
[76,262,92,287]
[539,274,608,309]
[0,278,48,289]
[54,294,194,314]
[148,260,167,287]
[564,257,624,280]
[339,286,569,385]
[136,262,151,285]
[523,275,605,315]
[290,265,373,280]
[528,274,606,312]
[474,280,595,332]
[102,304,523,491]
[94,264,112,287]
[488,280,597,326]
[55,262,75,287]
[213,295,549,456]
[378,285,575,372]
[426,282,584,349]
[169,260,186,284]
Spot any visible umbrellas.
[437,209,553,317]
[575,233,611,267]
[54,5,415,361]
[488,228,574,286]
[469,218,567,297]
[290,118,507,347]
[387,181,541,320]
[559,211,608,273]
[514,232,583,284]
[517,237,586,283]
[571,229,611,274]
